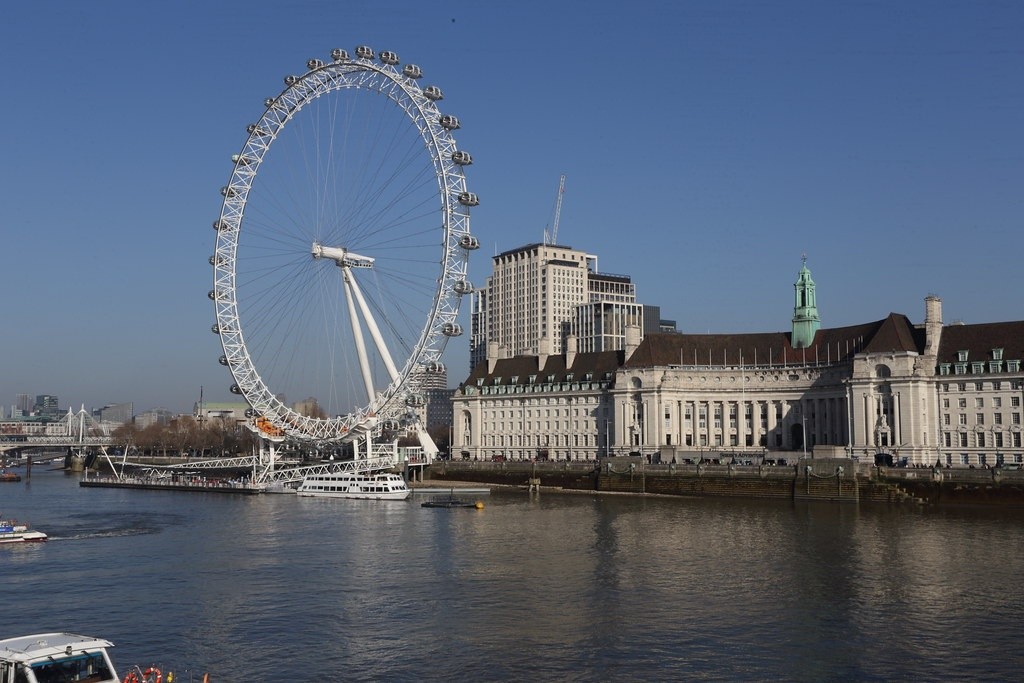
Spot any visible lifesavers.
[141,667,163,683]
[123,672,139,683]
